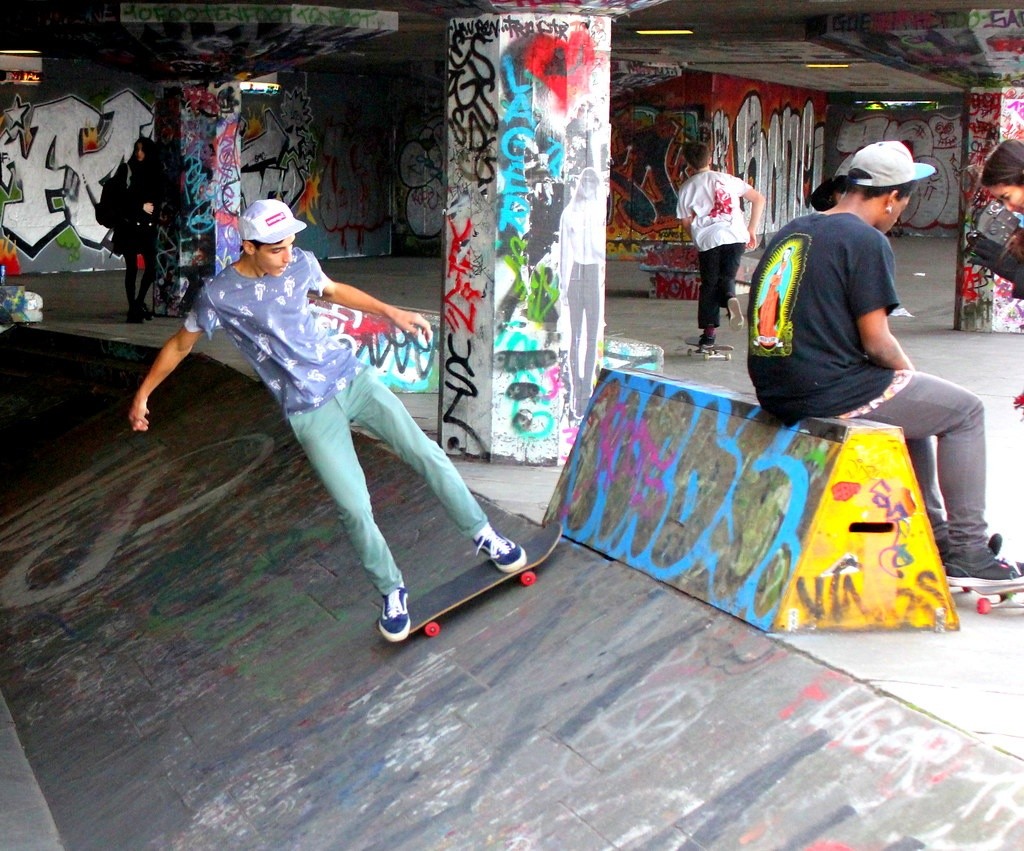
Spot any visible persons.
[126,199,527,643]
[746,141,1023,588]
[112,137,171,323]
[675,141,764,353]
[963,141,1023,300]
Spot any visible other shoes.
[726,297,744,331]
[699,332,715,348]
[127,302,152,323]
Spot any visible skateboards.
[675,334,734,364]
[950,576,1024,614]
[370,518,565,645]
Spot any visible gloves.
[970,239,1019,283]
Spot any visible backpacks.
[95,162,132,230]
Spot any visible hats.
[848,140,936,187]
[237,199,307,244]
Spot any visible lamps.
[635,27,697,35]
[805,63,850,67]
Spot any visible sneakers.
[472,521,527,573]
[945,534,1024,586]
[379,581,410,643]
